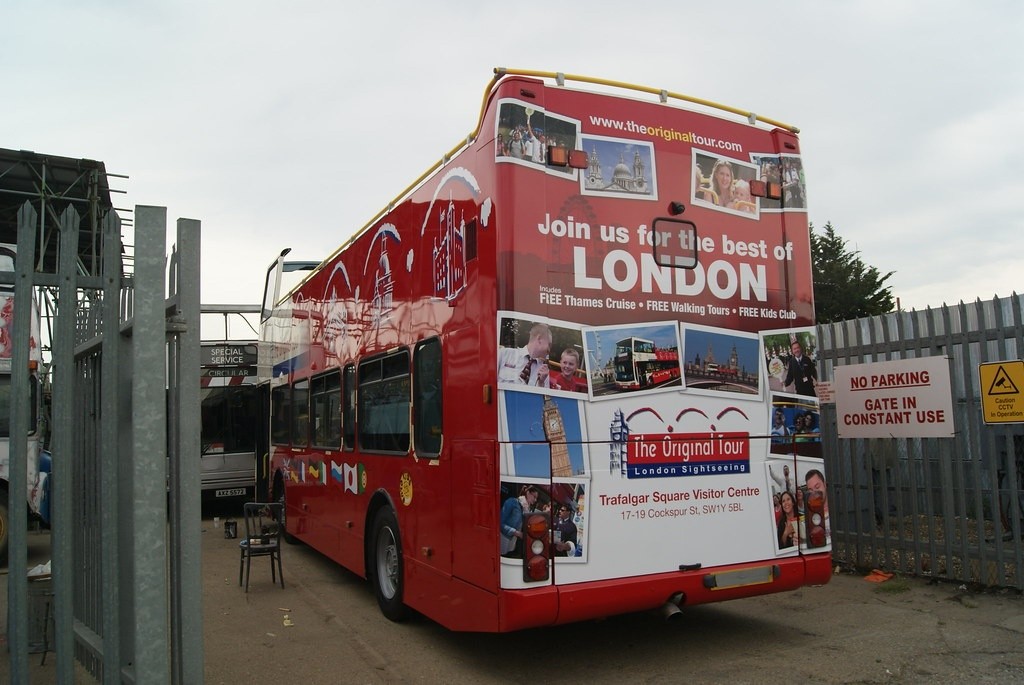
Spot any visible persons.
[552,348,580,392]
[769,463,831,549]
[500,484,577,559]
[693,158,756,215]
[772,408,821,445]
[497,115,574,174]
[764,340,818,396]
[760,158,807,209]
[497,322,553,390]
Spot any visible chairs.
[238,503,285,594]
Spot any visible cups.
[250,539,261,545]
[214,517,219,529]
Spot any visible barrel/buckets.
[224,516,237,540]
[7,568,55,654]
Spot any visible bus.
[166,339,258,502]
[255,68,834,633]
[0,242,49,552]
[310,372,413,436]
[615,337,681,390]
